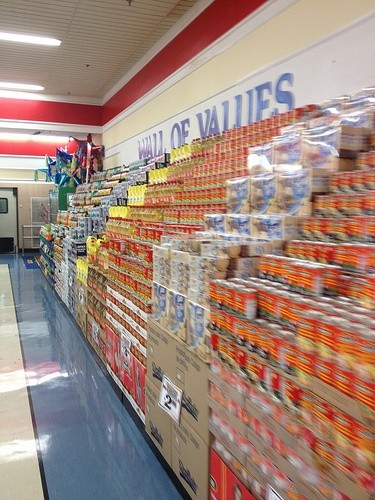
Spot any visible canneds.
[107,103,319,368]
[204,132,375,500]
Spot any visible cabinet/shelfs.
[22,224,42,257]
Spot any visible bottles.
[57,191,96,224]
[106,206,165,239]
[128,131,225,205]
[41,224,83,282]
[83,234,110,286]
[75,162,125,192]
